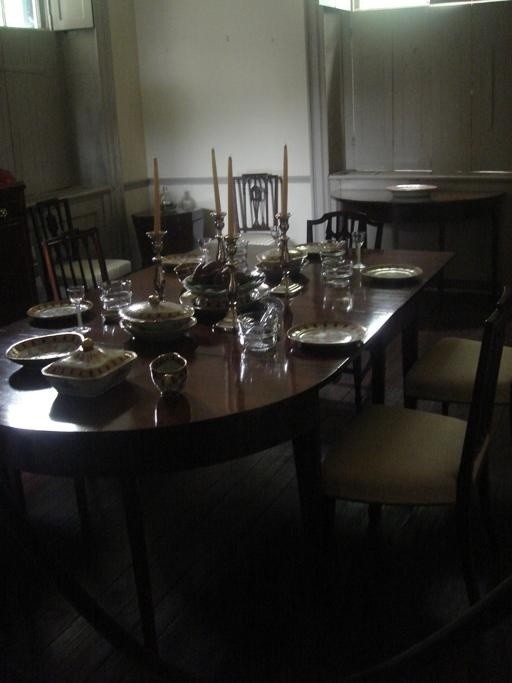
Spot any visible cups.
[320,249,346,280]
[237,310,279,353]
[100,277,131,311]
[149,352,188,401]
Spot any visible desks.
[331,188,507,328]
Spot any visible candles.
[154,147,288,236]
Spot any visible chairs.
[233,172,385,410]
[23,170,132,302]
[314,283,511,607]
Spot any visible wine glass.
[67,286,91,334]
[353,231,367,268]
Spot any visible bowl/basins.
[255,249,305,268]
[118,294,194,333]
[41,338,137,400]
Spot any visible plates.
[6,332,83,365]
[297,242,343,255]
[27,298,94,322]
[118,320,198,337]
[385,182,437,194]
[286,319,367,345]
[252,259,311,273]
[359,263,424,280]
[160,253,202,268]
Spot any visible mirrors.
[303,0,512,182]
